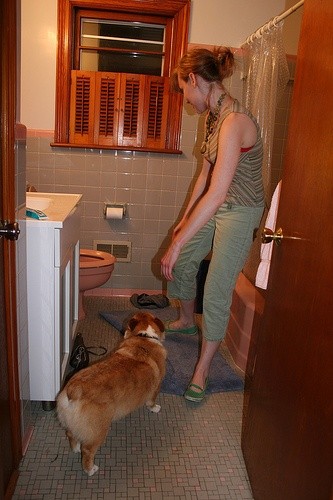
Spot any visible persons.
[153,44,265,402]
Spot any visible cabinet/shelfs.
[55,245,81,396]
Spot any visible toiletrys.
[25,208,49,220]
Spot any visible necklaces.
[200,90,226,154]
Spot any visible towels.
[255,178,282,287]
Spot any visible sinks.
[25,196,51,212]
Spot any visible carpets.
[96,306,247,395]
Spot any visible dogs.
[56,311,169,477]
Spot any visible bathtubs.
[225,271,272,375]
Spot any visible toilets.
[78,248,116,320]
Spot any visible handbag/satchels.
[69,333,89,369]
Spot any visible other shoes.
[163,319,198,336]
[184,376,210,403]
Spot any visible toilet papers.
[105,207,124,219]
